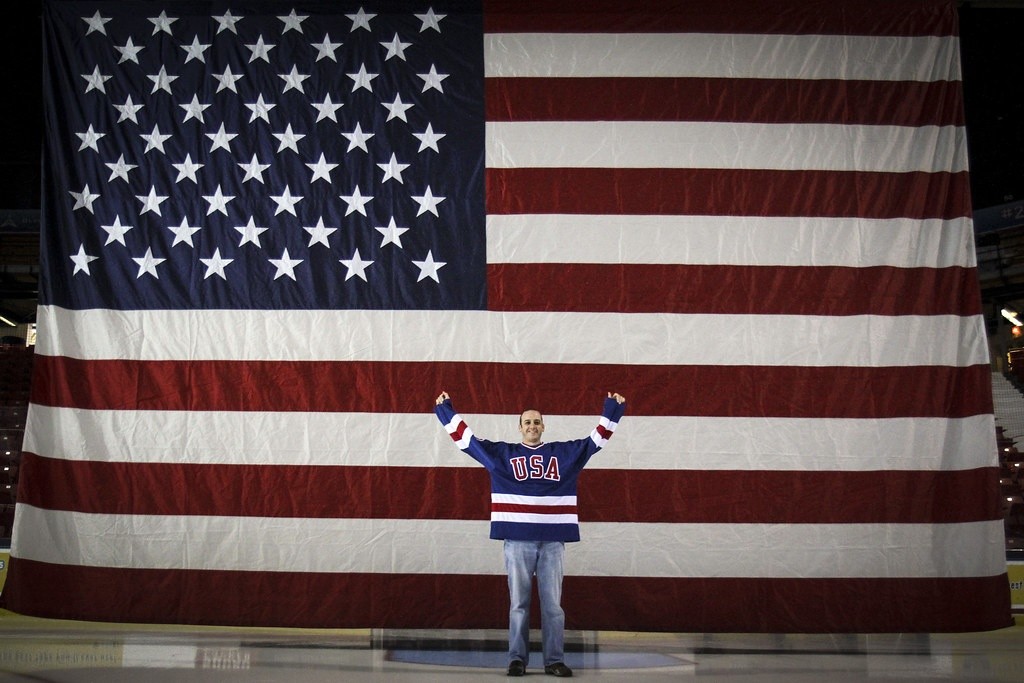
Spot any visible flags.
[0,0,1018,633]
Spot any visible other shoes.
[507,660,526,676]
[545,662,572,677]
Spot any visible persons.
[435,391,625,677]
